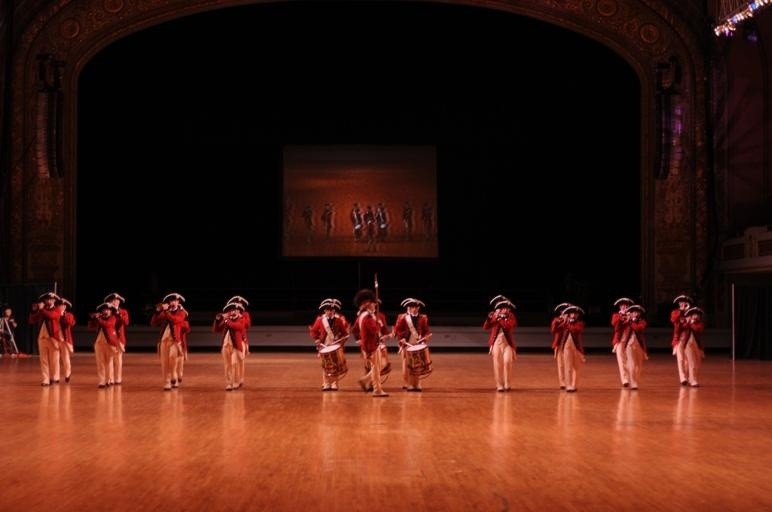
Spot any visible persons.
[308,292,431,397]
[151,293,190,390]
[670,295,704,387]
[0,309,17,353]
[481,295,517,392]
[91,293,128,388]
[284,199,433,252]
[552,303,586,392]
[213,296,251,391]
[612,298,649,389]
[33,292,76,386]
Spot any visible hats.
[489,293,705,318]
[39,292,248,312]
[319,293,426,315]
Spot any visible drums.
[319,344,348,377]
[363,345,392,375]
[406,343,432,376]
